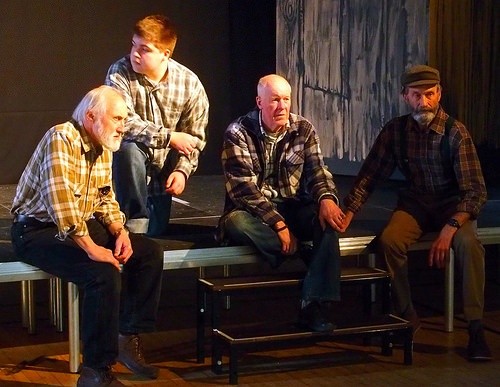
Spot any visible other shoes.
[297,300,336,332]
[465,327,493,361]
[395,311,422,344]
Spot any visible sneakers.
[117,334,159,380]
[77,365,126,387]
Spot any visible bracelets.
[276,225,288,233]
[319,195,336,201]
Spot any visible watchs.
[446,217,461,231]
[112,225,128,239]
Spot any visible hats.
[399,65,440,88]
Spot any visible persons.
[341,64,493,360]
[9,85,161,387]
[219,74,346,331]
[105,14,210,237]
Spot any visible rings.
[340,218,342,221]
